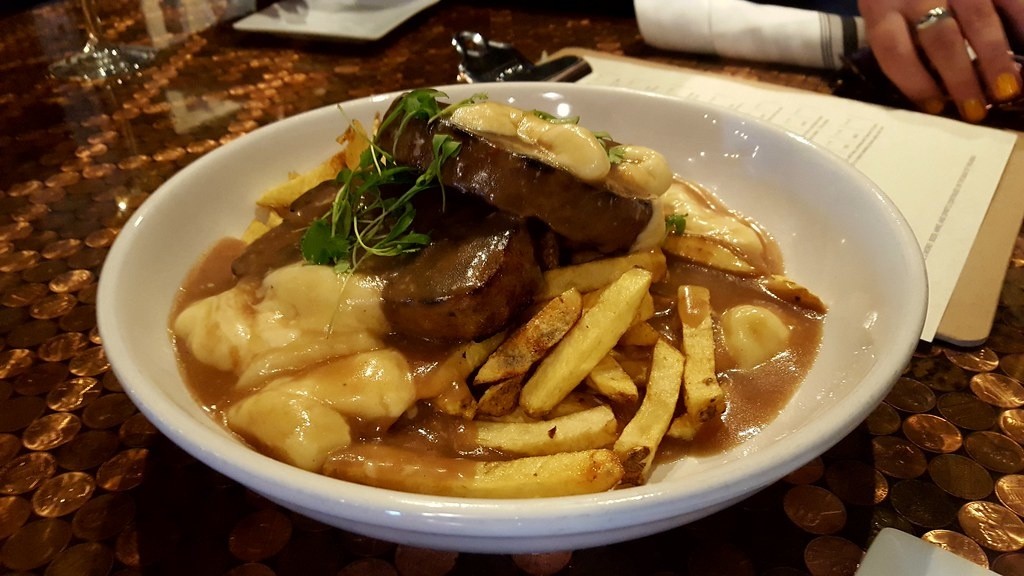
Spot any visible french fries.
[323,254,724,499]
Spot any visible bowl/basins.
[96,80,929,553]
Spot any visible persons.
[857,0,1024,122]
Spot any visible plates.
[232,0,440,41]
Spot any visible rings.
[917,6,947,29]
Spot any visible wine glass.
[48,0,169,79]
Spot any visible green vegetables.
[300,85,618,280]
